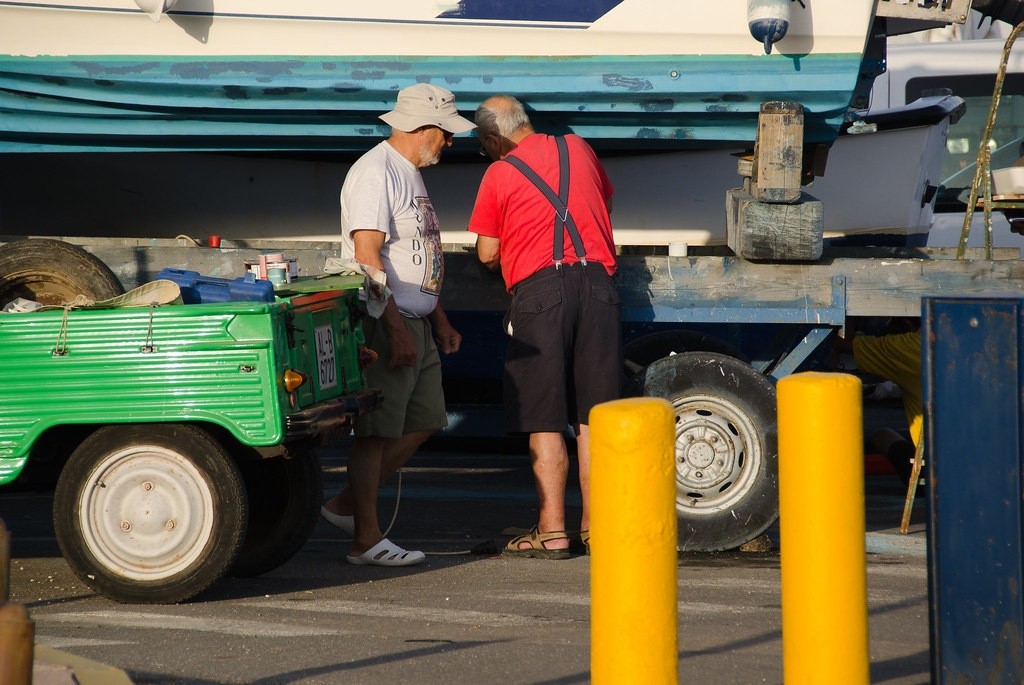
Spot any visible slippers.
[350,537,425,566]
[320,506,356,539]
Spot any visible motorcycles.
[1,234,372,608]
[0,0,1023,563]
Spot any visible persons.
[321,82,462,567]
[466,93,622,562]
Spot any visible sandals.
[566,528,590,556]
[500,525,571,560]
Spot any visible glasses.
[432,125,454,140]
[477,136,487,156]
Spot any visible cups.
[669,243,687,256]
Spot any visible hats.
[378,83,477,133]
